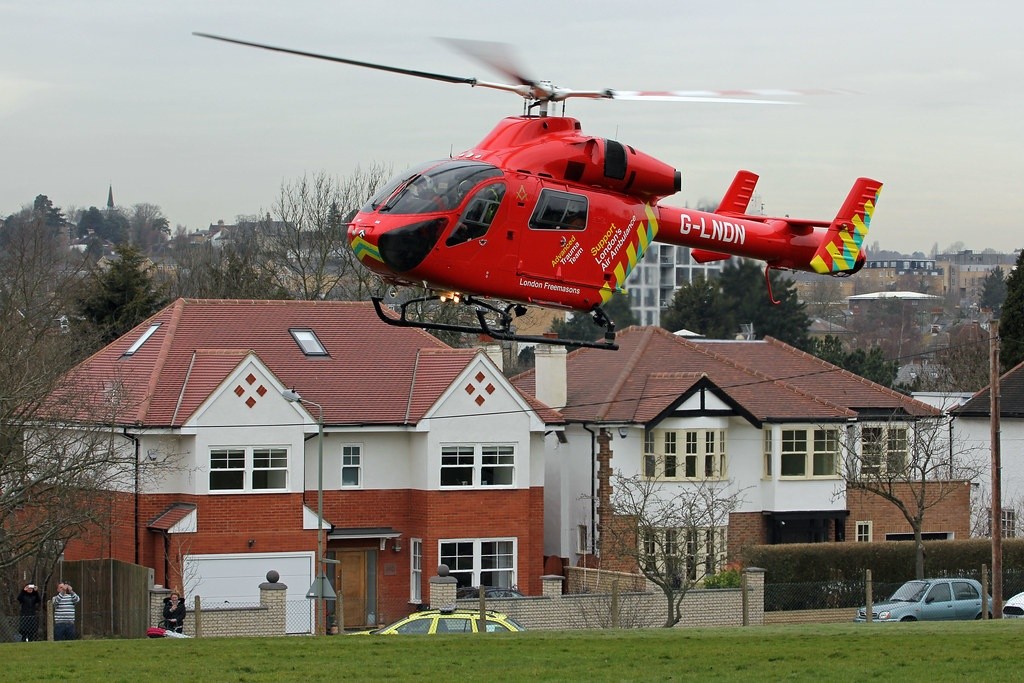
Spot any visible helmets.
[413,175,433,192]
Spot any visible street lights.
[282,387,325,636]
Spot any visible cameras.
[28,584,34,588]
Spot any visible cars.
[345,608,525,634]
[854,576,1024,623]
[455,585,525,599]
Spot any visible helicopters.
[189,27,885,353]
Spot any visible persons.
[52,582,81,642]
[469,179,499,228]
[16,582,42,642]
[163,591,186,636]
